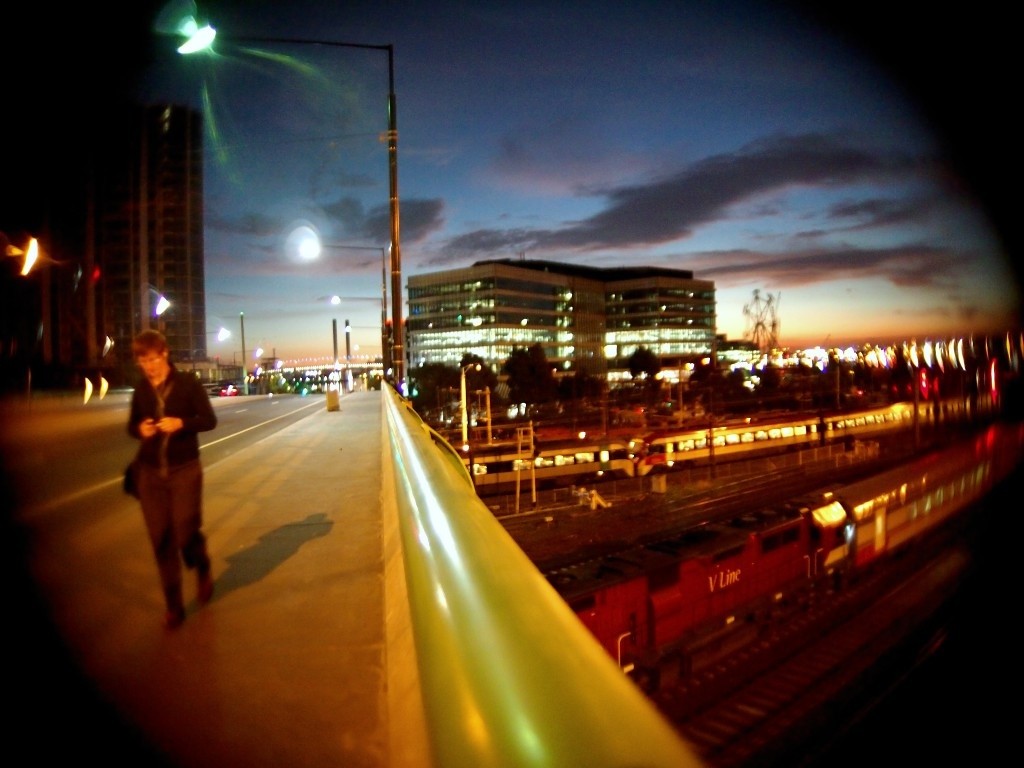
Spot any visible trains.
[454,389,1004,496]
[537,420,1023,675]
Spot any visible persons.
[126,331,218,630]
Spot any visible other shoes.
[196,558,213,603]
[165,607,185,625]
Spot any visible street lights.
[459,362,482,445]
[331,295,385,370]
[187,26,406,392]
[302,241,389,380]
